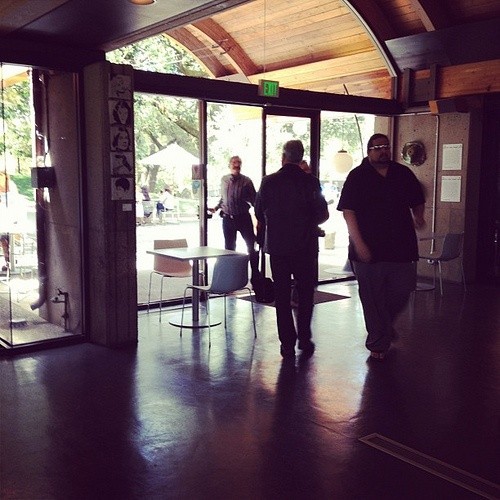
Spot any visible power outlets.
[122,203,133,212]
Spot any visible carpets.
[238,289,350,308]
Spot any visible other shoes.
[391,328,398,343]
[281,345,295,356]
[299,341,315,351]
[370,352,385,361]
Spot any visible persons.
[141,184,151,217]
[156,188,175,218]
[111,127,130,151]
[292,161,325,301]
[0,155,21,269]
[206,156,257,286]
[114,177,131,200]
[336,132,428,358]
[114,152,132,173]
[111,101,131,125]
[255,141,330,363]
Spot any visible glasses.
[370,145,391,151]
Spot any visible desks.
[151,198,162,223]
[147,245,246,327]
[2,226,25,275]
[416,230,444,291]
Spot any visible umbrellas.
[137,142,202,192]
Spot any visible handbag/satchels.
[250,272,275,303]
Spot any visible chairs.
[420,233,467,297]
[0,209,38,285]
[146,239,208,323]
[136,192,179,226]
[180,255,257,350]
